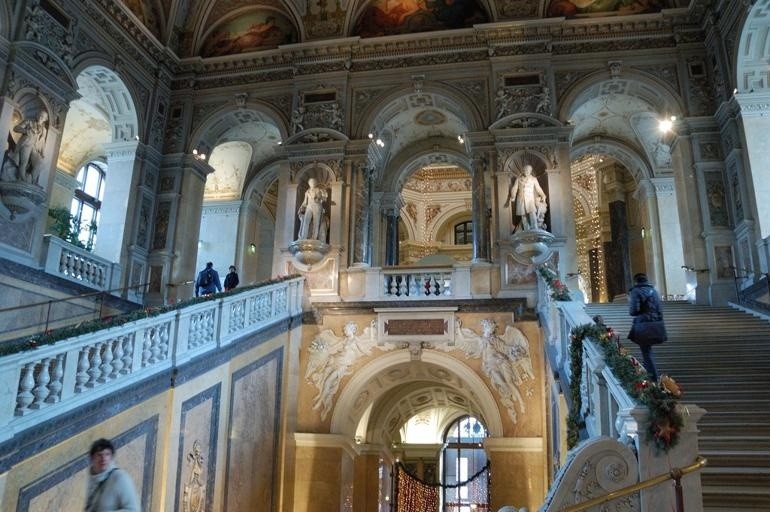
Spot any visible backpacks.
[198,268,213,285]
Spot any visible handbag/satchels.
[632,320,667,344]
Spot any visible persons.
[195,262,222,297]
[14,110,49,185]
[224,265,239,294]
[628,272,668,382]
[81,438,140,511]
[320,103,343,131]
[495,88,513,120]
[533,86,553,116]
[297,177,328,240]
[479,317,526,413]
[310,319,375,410]
[509,164,546,229]
[291,107,304,135]
